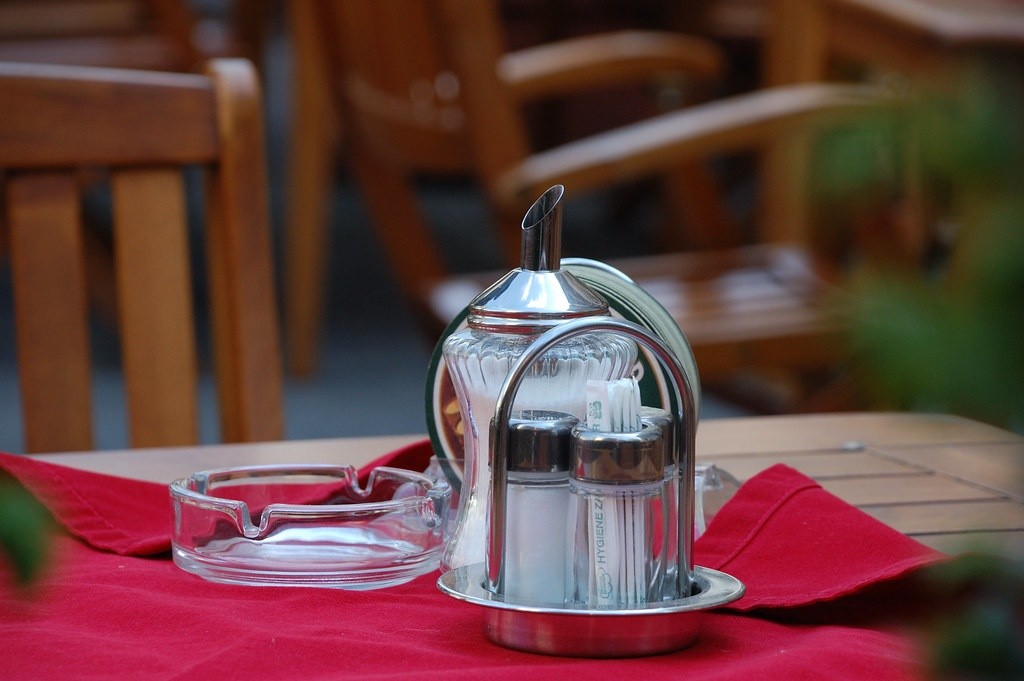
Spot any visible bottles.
[438,183,638,598]
[566,418,668,609]
[486,409,577,608]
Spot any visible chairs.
[1,1,899,455]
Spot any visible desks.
[757,1,1024,418]
[0,415,1024,681]
